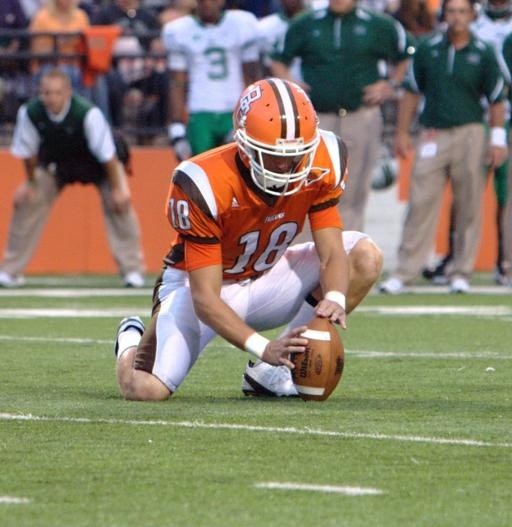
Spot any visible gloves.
[171,135,192,162]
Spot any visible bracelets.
[322,288,348,311]
[490,127,506,146]
[168,120,186,140]
[242,330,272,360]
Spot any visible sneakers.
[113,316,146,357]
[241,354,299,398]
[448,275,471,294]
[121,270,144,288]
[377,276,404,296]
[0,268,25,289]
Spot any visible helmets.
[229,75,322,198]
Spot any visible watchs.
[386,77,398,88]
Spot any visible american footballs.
[291,316,344,400]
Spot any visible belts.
[311,102,364,117]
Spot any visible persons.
[3,68,148,288]
[161,1,261,162]
[259,1,417,248]
[2,1,172,147]
[380,2,511,292]
[392,1,434,134]
[115,77,387,401]
[157,1,198,26]
